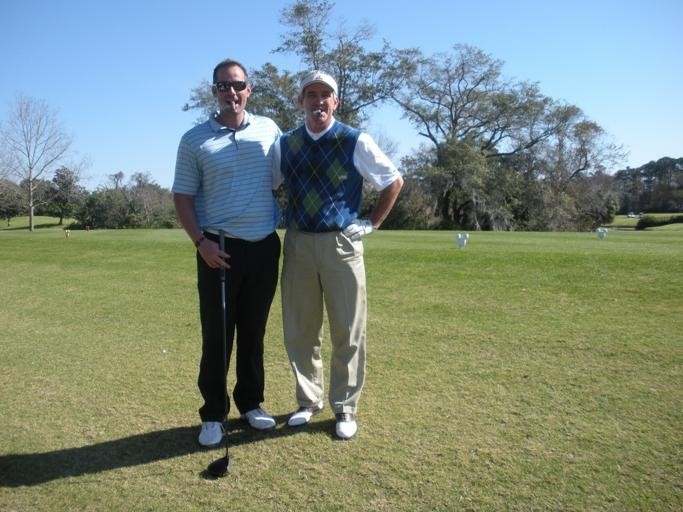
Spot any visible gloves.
[343,218,374,241]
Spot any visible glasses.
[213,80,248,92]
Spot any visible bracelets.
[194,236,206,248]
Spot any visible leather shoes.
[336,412,357,438]
[287,399,323,427]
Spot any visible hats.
[300,70,338,97]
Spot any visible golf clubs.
[208,230,229,477]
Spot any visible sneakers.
[198,422,223,446]
[240,406,276,430]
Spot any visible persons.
[172,60,283,448]
[277,70,404,440]
